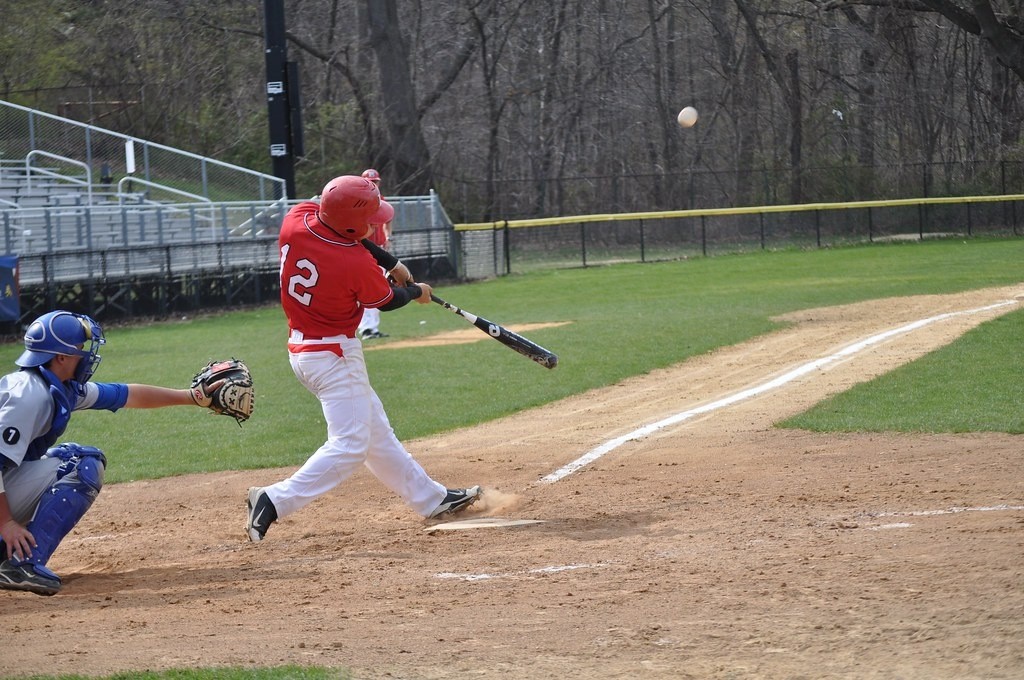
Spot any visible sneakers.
[359,330,376,341]
[243,485,277,543]
[371,328,389,338]
[0,557,61,598]
[429,483,482,521]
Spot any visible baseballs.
[677,107,698,128]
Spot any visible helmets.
[318,175,394,239]
[14,309,88,368]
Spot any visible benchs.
[0,152,215,242]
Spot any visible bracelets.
[386,236,394,243]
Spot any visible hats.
[358,169,381,182]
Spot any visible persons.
[245,173,483,542]
[355,168,394,342]
[0,307,254,596]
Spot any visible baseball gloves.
[190,358,254,423]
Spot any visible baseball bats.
[385,271,559,370]
